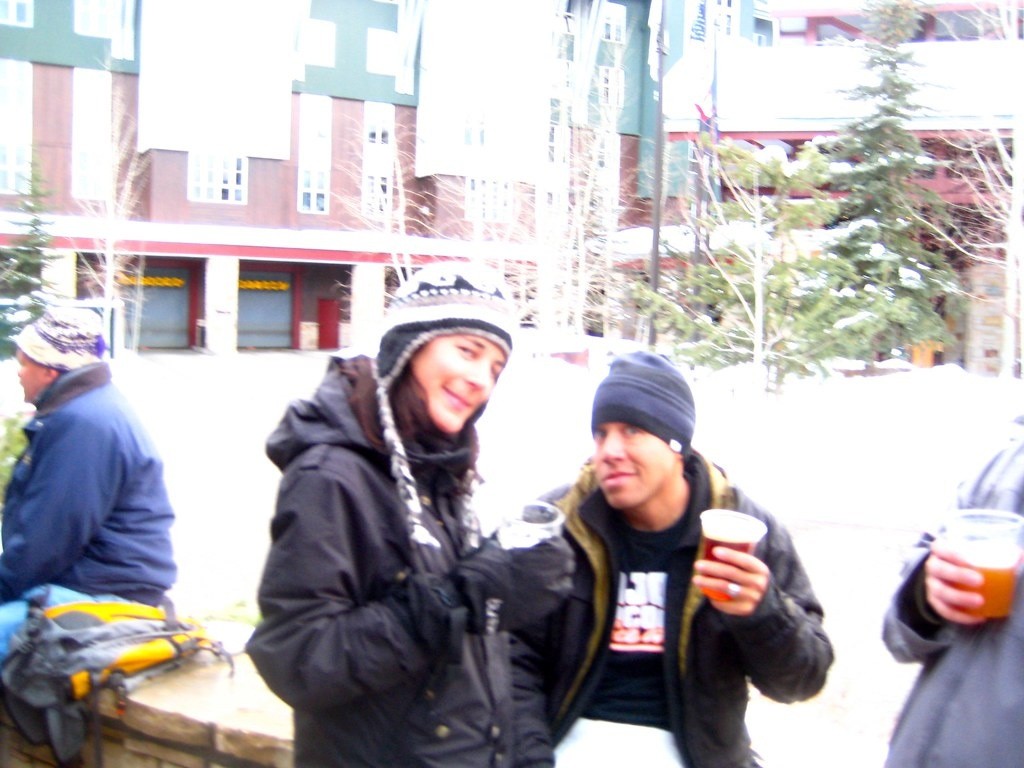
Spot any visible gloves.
[449,528,578,637]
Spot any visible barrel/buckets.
[298,322,319,350]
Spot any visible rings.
[727,582,740,597]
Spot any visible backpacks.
[0,585,234,768]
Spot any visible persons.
[243,261,834,768]
[882,406,1024,766]
[0,299,177,768]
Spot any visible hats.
[13,306,107,373]
[371,260,517,552]
[591,350,696,456]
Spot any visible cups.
[500,502,564,549]
[701,509,768,600]
[946,509,1024,618]
